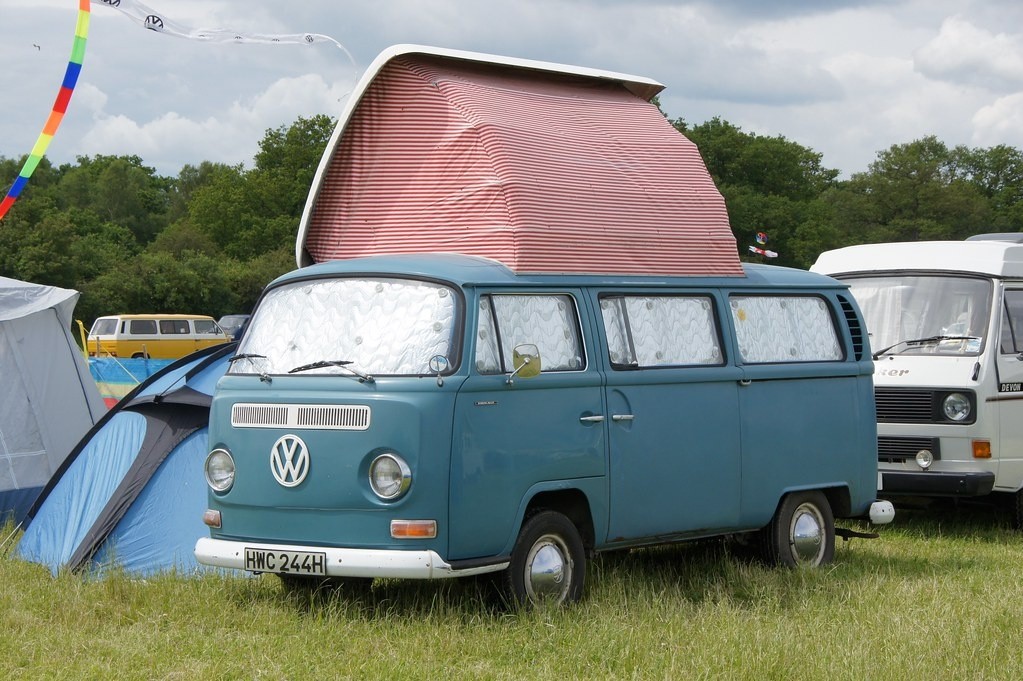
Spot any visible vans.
[208,315,252,341]
[86,313,230,364]
[191,45,896,629]
[804,231,1023,528]
